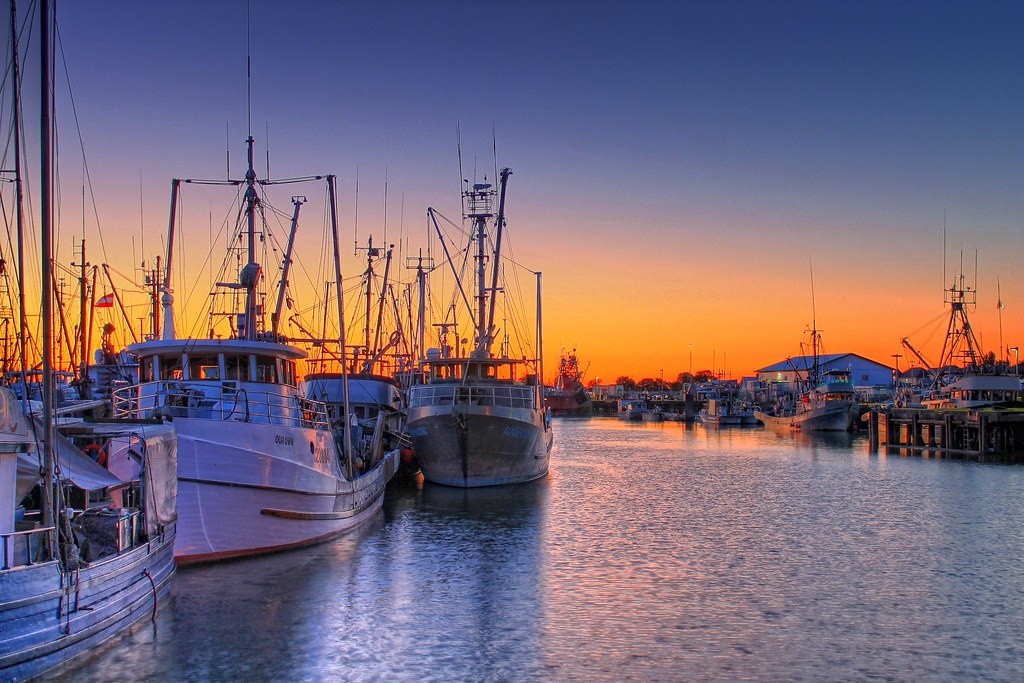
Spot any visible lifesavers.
[82,444,106,465]
[390,331,401,346]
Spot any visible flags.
[95,293,114,307]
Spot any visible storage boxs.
[14,521,42,533]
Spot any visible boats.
[544,345,593,417]
[0,0,553,683]
[617,398,650,421]
[754,254,861,431]
[698,348,771,424]
[861,206,1024,445]
